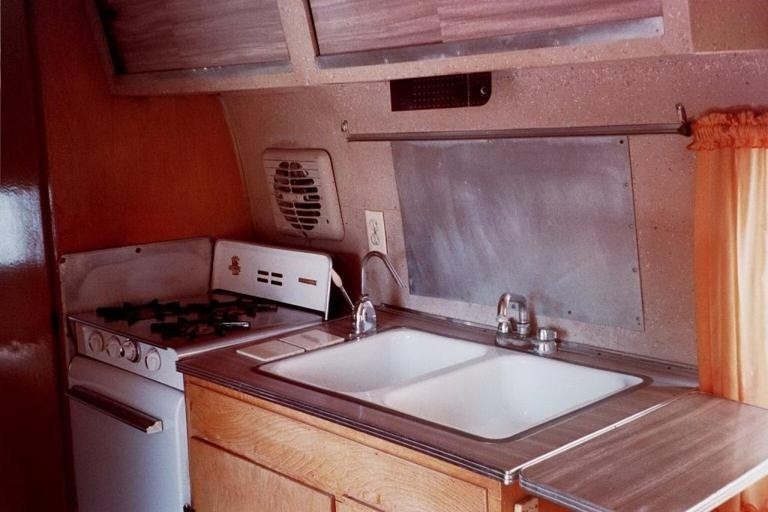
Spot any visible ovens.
[48,362,190,510]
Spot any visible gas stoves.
[69,292,313,389]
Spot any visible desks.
[518,391,768,512]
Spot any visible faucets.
[496,291,559,353]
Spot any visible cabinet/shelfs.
[182,373,524,512]
[74,0,767,99]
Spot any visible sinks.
[252,324,496,400]
[366,347,653,442]
